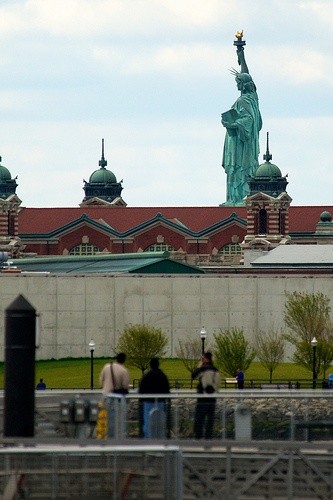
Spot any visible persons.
[138,357,171,438]
[36,379,46,390]
[219,67,262,207]
[190,352,220,439]
[236,369,333,389]
[99,353,130,439]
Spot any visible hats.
[204,352,212,360]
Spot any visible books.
[222,108,241,136]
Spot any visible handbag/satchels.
[113,388,129,403]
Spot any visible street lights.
[199,325,206,359]
[310,336,319,388]
[88,336,97,391]
